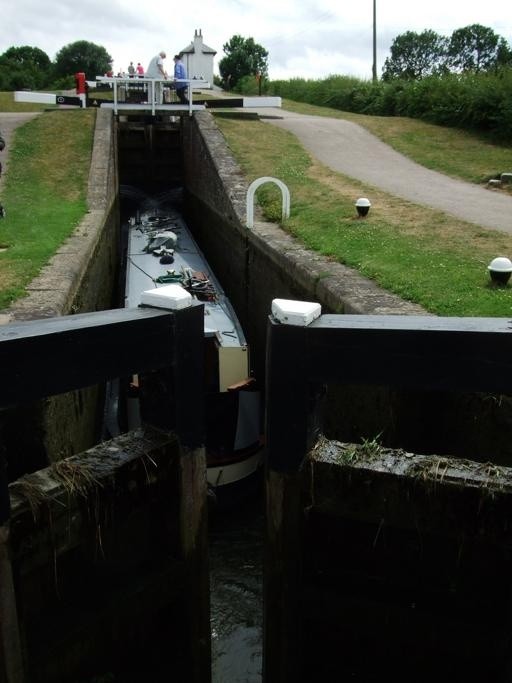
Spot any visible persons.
[135,62,145,78]
[145,51,168,101]
[127,62,135,78]
[172,55,189,104]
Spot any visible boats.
[102,198,264,525]
